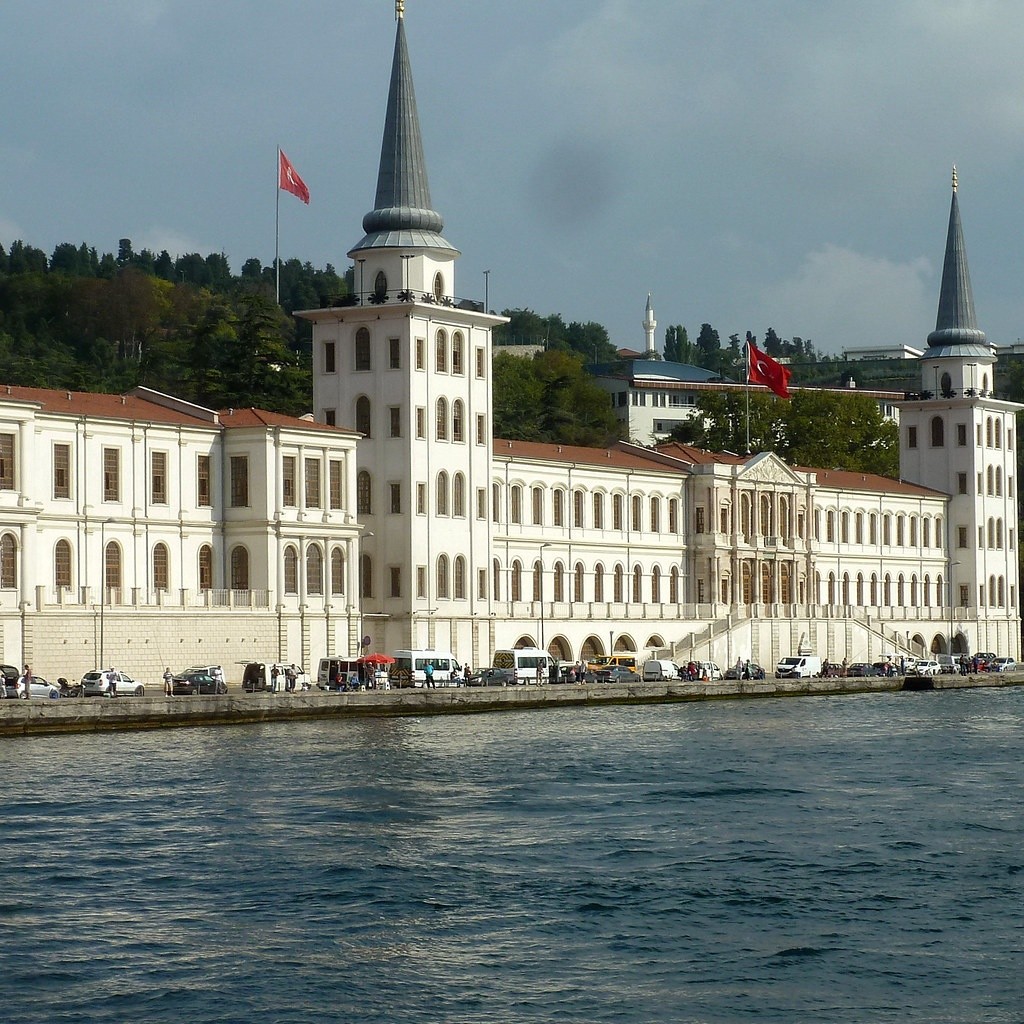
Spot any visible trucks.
[388,649,467,687]
[775,656,822,678]
[492,647,563,685]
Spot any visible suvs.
[80,669,145,697]
[184,664,227,685]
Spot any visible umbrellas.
[357,653,395,665]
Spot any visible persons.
[744,659,752,681]
[581,660,586,685]
[736,657,744,681]
[820,659,829,679]
[842,658,849,678]
[350,673,360,692]
[271,664,279,693]
[883,654,998,676]
[23,664,32,700]
[289,664,297,694]
[536,662,543,687]
[451,669,461,688]
[574,661,581,686]
[107,666,117,698]
[0,675,7,699]
[215,666,222,695]
[363,662,375,691]
[163,667,174,697]
[464,667,472,688]
[678,662,701,682]
[424,661,436,689]
[335,673,348,692]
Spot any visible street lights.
[540,542,553,650]
[359,530,375,655]
[950,561,962,652]
[99,515,119,672]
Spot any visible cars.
[595,666,641,683]
[873,662,903,677]
[822,664,845,678]
[163,672,228,695]
[0,665,60,699]
[724,663,766,680]
[465,667,508,688]
[550,665,598,683]
[847,663,878,677]
[906,652,1017,676]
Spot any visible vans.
[587,654,636,673]
[642,660,682,682]
[315,655,388,692]
[234,660,312,692]
[693,660,724,681]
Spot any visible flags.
[277,147,309,205]
[745,341,791,398]
